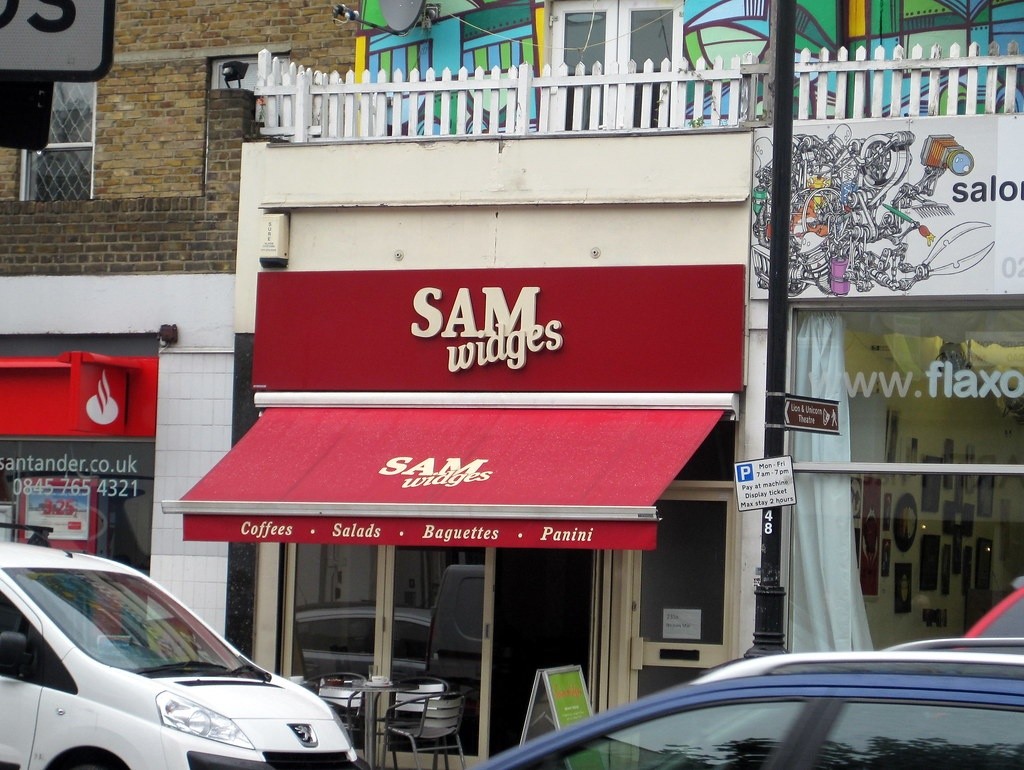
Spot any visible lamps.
[222,61,249,89]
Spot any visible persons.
[881,542,890,576]
[894,574,912,613]
[861,507,879,595]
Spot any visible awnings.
[161,409,740,549]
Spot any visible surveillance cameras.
[222,67,233,76]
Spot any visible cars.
[468,582,1024,770]
[291,601,434,681]
[0,523,370,770]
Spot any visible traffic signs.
[784,393,842,436]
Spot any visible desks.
[317,678,419,770]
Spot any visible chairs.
[305,671,468,770]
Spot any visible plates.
[365,682,393,687]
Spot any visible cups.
[371,676,389,683]
